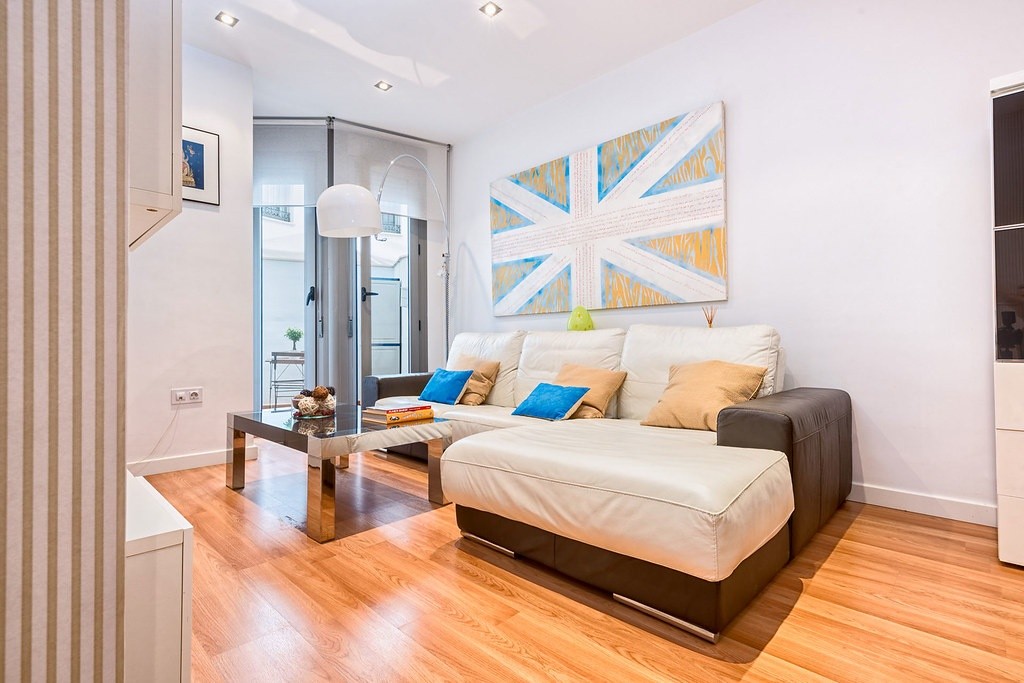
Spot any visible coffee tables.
[225,401,453,544]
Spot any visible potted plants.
[284,328,303,350]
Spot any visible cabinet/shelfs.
[123,470,193,683]
[988,67,1024,569]
[127,0,182,252]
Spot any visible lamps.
[315,152,450,361]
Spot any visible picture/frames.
[182,125,220,207]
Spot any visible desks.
[271,352,304,413]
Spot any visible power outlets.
[171,387,203,405]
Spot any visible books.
[361,402,435,425]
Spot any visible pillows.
[513,328,628,418]
[639,359,768,431]
[446,329,527,408]
[417,367,474,405]
[617,322,781,420]
[511,382,591,421]
[452,353,501,406]
[553,363,628,419]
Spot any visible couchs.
[361,346,853,645]
